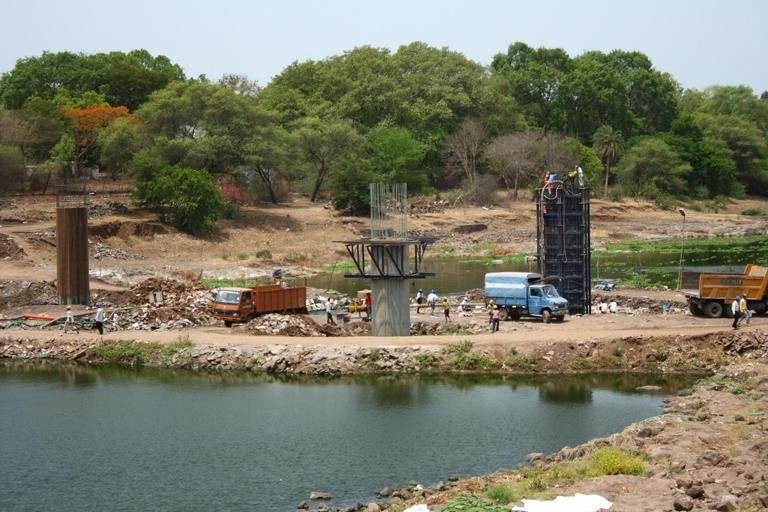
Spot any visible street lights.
[678,208,688,267]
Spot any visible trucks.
[483,271,569,323]
[679,262,768,318]
[212,283,308,329]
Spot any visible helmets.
[66,305,71,310]
[489,299,498,309]
[736,293,747,301]
[419,289,435,292]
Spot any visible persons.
[272,268,281,279]
[731,294,752,329]
[64,305,79,334]
[326,297,335,323]
[415,288,439,315]
[575,166,583,186]
[95,302,104,334]
[487,299,500,334]
[591,293,623,313]
[458,297,468,312]
[544,171,553,194]
[442,296,452,324]
[344,292,372,318]
[663,303,670,321]
